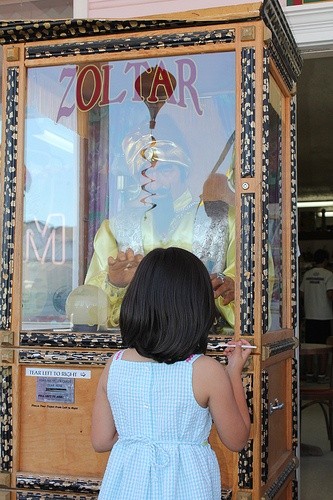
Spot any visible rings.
[217,271,225,282]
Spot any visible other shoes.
[318,374,326,383]
[305,373,314,383]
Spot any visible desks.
[301,342,333,457]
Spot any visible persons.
[88,246,253,500]
[298,248,333,377]
[83,128,275,339]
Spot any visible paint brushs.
[219,344,257,349]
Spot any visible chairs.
[300,336,333,452]
